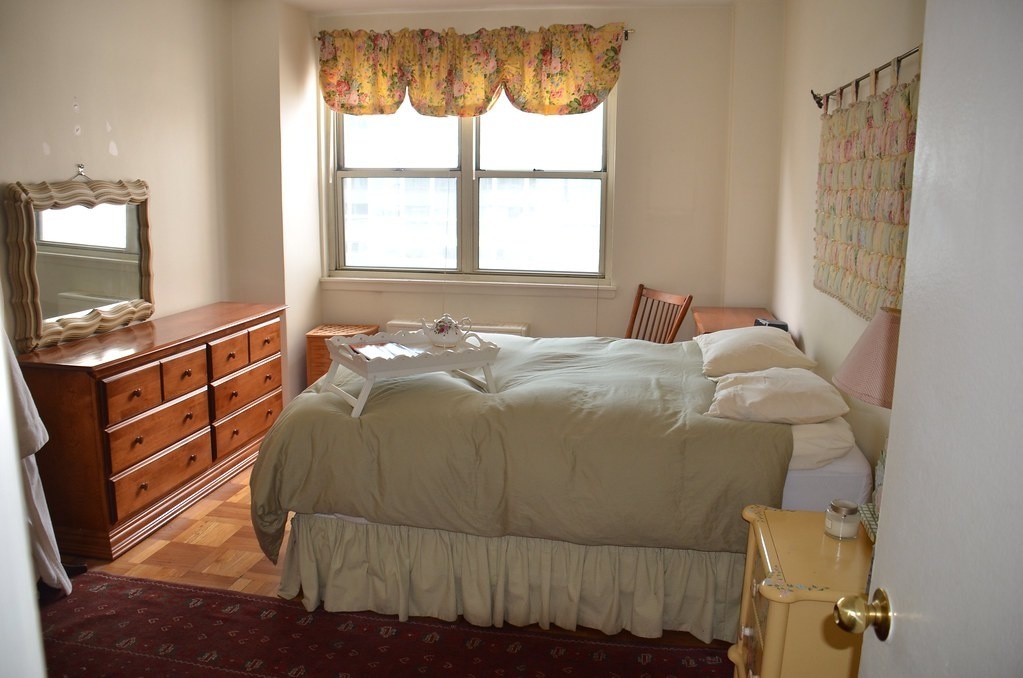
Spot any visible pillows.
[699,326,820,376]
[705,365,850,420]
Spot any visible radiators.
[386,320,531,338]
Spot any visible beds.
[249,330,875,646]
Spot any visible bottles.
[823,499,861,541]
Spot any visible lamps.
[829,306,901,409]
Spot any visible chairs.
[624,284,693,344]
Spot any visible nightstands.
[692,304,777,340]
[728,503,873,678]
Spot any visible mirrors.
[5,178,154,353]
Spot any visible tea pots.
[419,313,472,348]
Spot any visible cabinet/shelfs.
[305,324,381,390]
[15,300,293,564]
